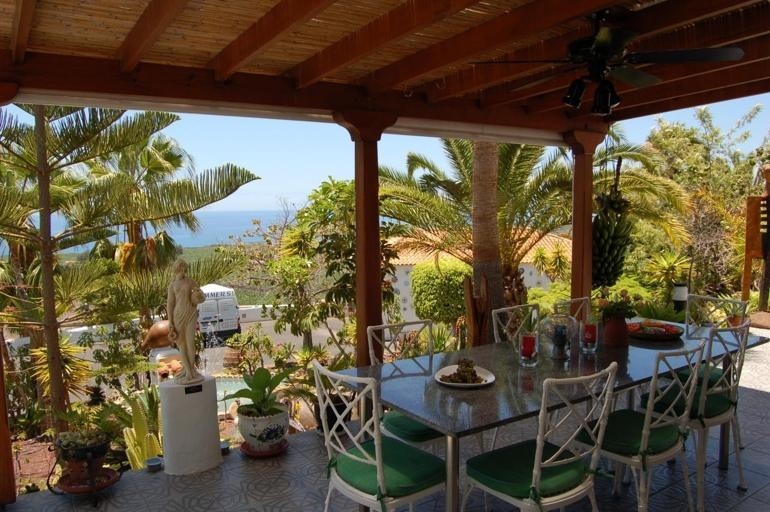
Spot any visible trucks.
[186,284,241,347]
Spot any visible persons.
[167,259,206,381]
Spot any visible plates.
[433,362,497,387]
[625,320,686,340]
[630,340,686,351]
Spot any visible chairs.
[651,293,749,452]
[554,296,620,424]
[326,355,448,511]
[567,340,705,511]
[640,319,752,511]
[367,319,484,478]
[458,360,622,511]
[493,304,541,452]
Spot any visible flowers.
[597,286,647,321]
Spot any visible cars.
[6,335,32,356]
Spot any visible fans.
[469,14,746,116]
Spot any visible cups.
[516,365,540,402]
[578,317,600,353]
[512,328,541,367]
[578,352,597,377]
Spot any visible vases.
[602,316,627,352]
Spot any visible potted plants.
[217,362,301,463]
[50,401,124,507]
[288,343,356,437]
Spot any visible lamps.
[566,69,624,117]
[670,283,690,314]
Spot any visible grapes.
[440,359,483,383]
